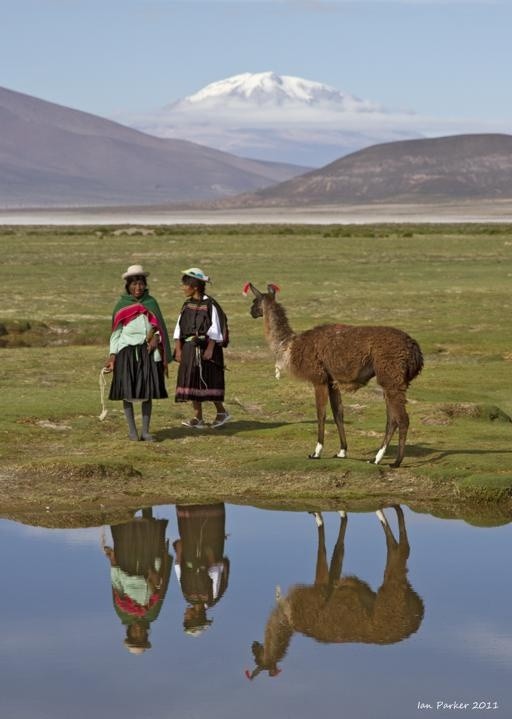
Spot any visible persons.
[169,502,235,638]
[173,266,232,430]
[102,262,173,443]
[98,504,173,656]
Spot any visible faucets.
[181,417,207,430]
[210,411,231,428]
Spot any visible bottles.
[122,639,151,654]
[181,267,210,284]
[122,264,150,280]
[184,623,211,638]
